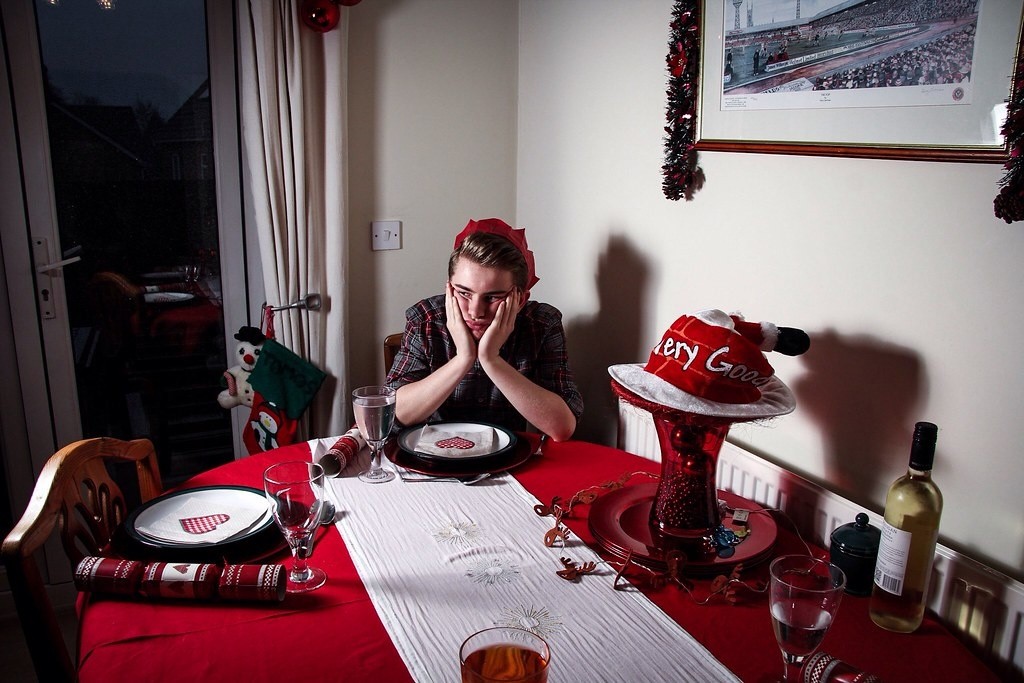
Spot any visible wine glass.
[352,386,396,484]
[264,462,325,593]
[768,555,846,683]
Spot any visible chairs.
[0,435,172,683]
[97,269,140,301]
[384,333,402,378]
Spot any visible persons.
[724,0,979,91]
[378,217,589,442]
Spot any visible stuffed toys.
[219,326,268,409]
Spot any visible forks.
[533,434,549,456]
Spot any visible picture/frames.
[689,0,1023,165]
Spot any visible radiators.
[614,400,1024,682]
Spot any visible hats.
[454,218,540,291]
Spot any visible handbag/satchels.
[240,307,325,458]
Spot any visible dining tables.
[116,280,221,355]
[82,429,1006,683]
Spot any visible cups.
[458,627,551,683]
[185,265,200,282]
[829,513,882,596]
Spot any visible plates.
[111,497,309,564]
[382,432,533,476]
[586,482,778,577]
[142,292,194,305]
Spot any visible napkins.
[413,424,494,457]
[136,496,267,543]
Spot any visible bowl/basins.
[397,420,518,470]
[127,485,281,557]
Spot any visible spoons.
[401,473,491,485]
[298,499,335,558]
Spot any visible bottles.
[868,422,954,633]
